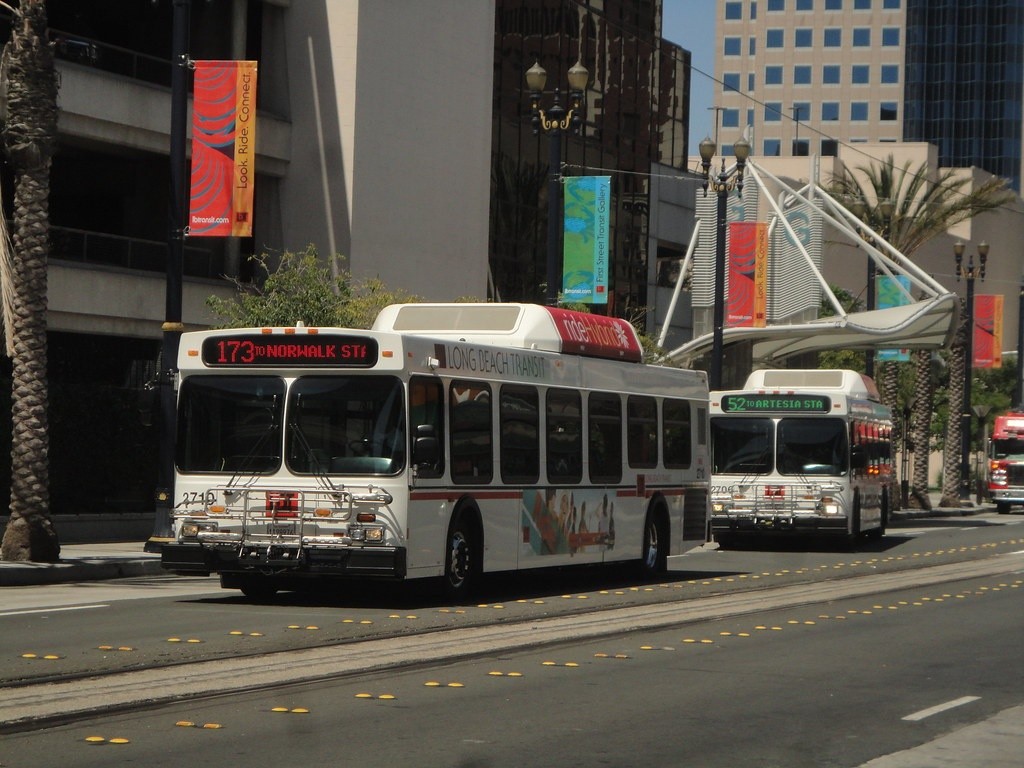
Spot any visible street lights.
[952,235,993,498]
[695,133,751,391]
[524,54,592,309]
[851,194,897,383]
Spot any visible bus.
[158,298,714,606]
[707,369,895,554]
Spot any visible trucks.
[986,409,1023,514]
